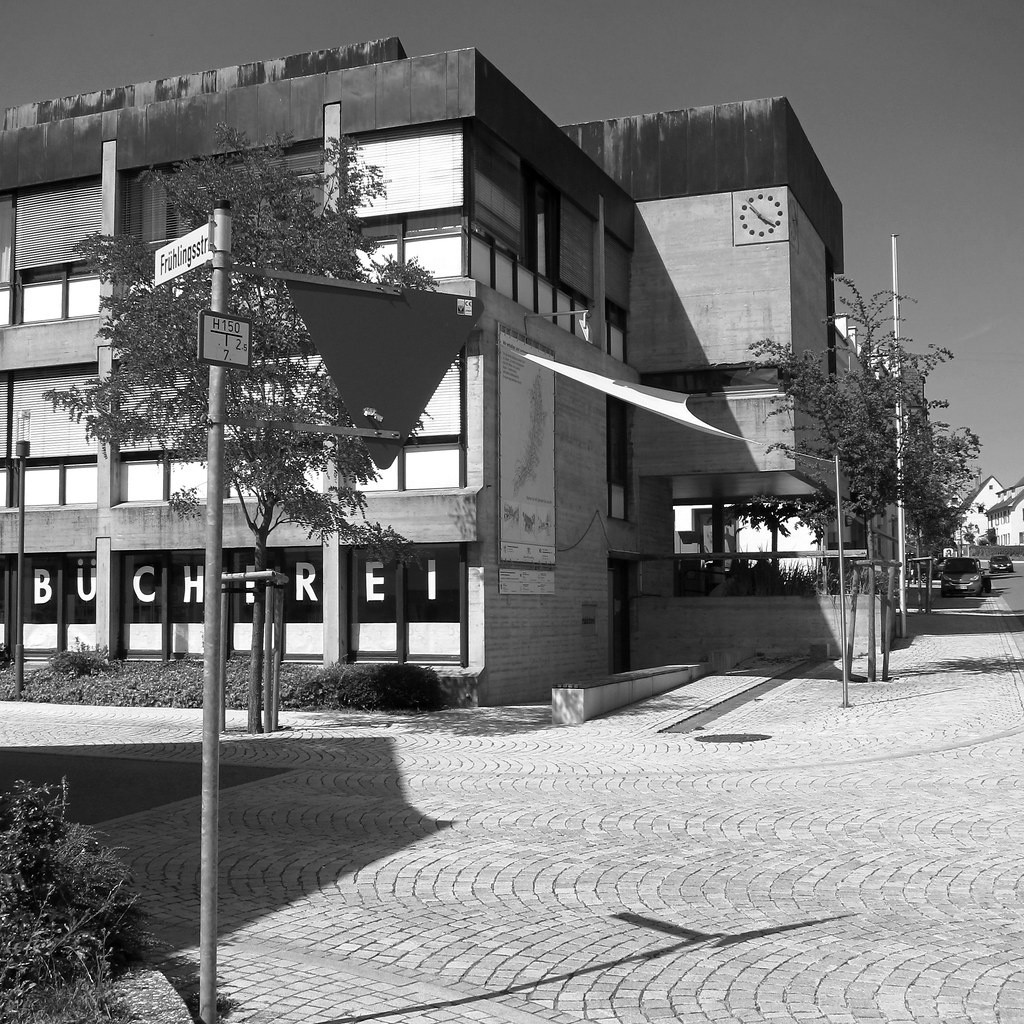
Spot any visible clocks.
[788,189,800,252]
[731,186,789,246]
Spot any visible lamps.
[523,310,594,344]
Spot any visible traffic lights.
[979,507,983,513]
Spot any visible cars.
[941,557,982,597]
[910,563,943,581]
[988,555,1014,574]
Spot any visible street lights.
[15,410,31,702]
[959,521,962,557]
